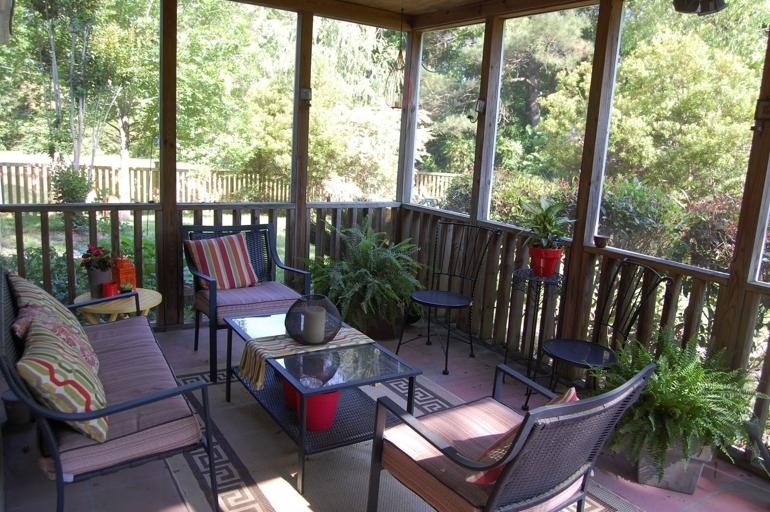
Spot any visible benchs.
[0,255,220,511]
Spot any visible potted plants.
[510,187,571,276]
[299,207,436,341]
[587,325,763,495]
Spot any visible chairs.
[394,218,502,377]
[365,362,658,512]
[180,224,310,381]
[523,258,674,411]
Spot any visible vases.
[88,268,113,298]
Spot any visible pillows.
[17,319,111,441]
[10,271,101,372]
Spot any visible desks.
[224,309,417,493]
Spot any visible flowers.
[81,245,114,272]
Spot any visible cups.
[102,281,118,297]
[303,306,326,344]
